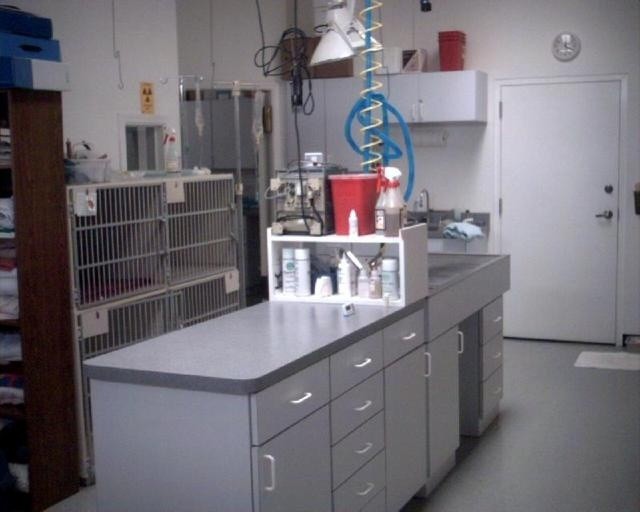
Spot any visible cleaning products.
[374,177,388,236]
[376,163,408,237]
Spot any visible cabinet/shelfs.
[266,224,430,309]
[1,81,83,511]
[331,330,388,511]
[460,293,509,422]
[425,320,461,498]
[86,356,334,512]
[387,308,430,507]
[283,77,368,174]
[370,68,490,127]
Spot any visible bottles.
[295,251,312,294]
[279,248,296,291]
[332,249,400,301]
[164,126,181,171]
[347,208,360,241]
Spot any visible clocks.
[552,30,581,63]
